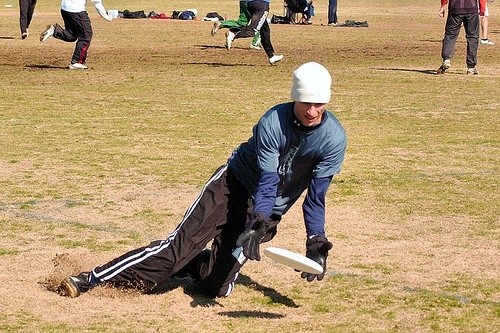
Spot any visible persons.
[328,0,338,25]
[59,61,349,299]
[19,0,37,39]
[477,0,495,44]
[226,0,284,64]
[437,0,487,75]
[286,0,313,24]
[210,0,261,50]
[40,0,112,70]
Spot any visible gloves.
[294,235,333,281]
[235,212,269,261]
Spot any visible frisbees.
[263,247,323,275]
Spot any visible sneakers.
[211,21,220,37]
[225,30,236,50]
[250,44,262,50]
[22,32,28,39]
[467,68,478,74]
[480,39,495,45]
[40,24,55,42]
[69,62,89,70]
[60,275,90,298]
[437,59,451,74]
[269,54,284,64]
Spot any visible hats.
[291,61,333,105]
